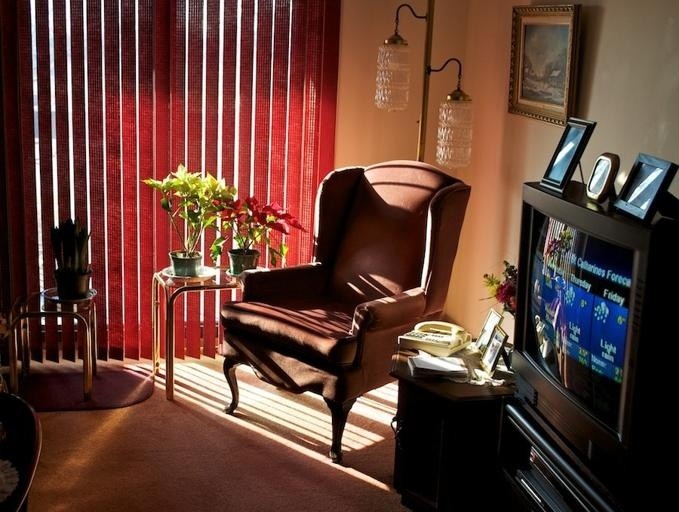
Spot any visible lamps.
[374,0,473,170]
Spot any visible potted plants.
[208,196,308,279]
[543,229,574,269]
[41,216,97,303]
[138,164,238,283]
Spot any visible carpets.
[2,364,154,412]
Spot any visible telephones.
[398,320,471,357]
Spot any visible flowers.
[482,262,518,319]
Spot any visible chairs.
[215,159,472,464]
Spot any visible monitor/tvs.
[510,180,679,508]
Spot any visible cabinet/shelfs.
[388,364,515,512]
[492,395,623,512]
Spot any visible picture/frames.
[539,117,597,199]
[475,307,505,356]
[507,3,583,129]
[586,152,620,205]
[478,325,510,376]
[613,152,679,226]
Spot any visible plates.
[41,288,95,303]
[163,267,216,281]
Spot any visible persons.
[528,272,571,390]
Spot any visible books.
[407,356,468,379]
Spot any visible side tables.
[151,266,242,401]
[9,291,97,397]
